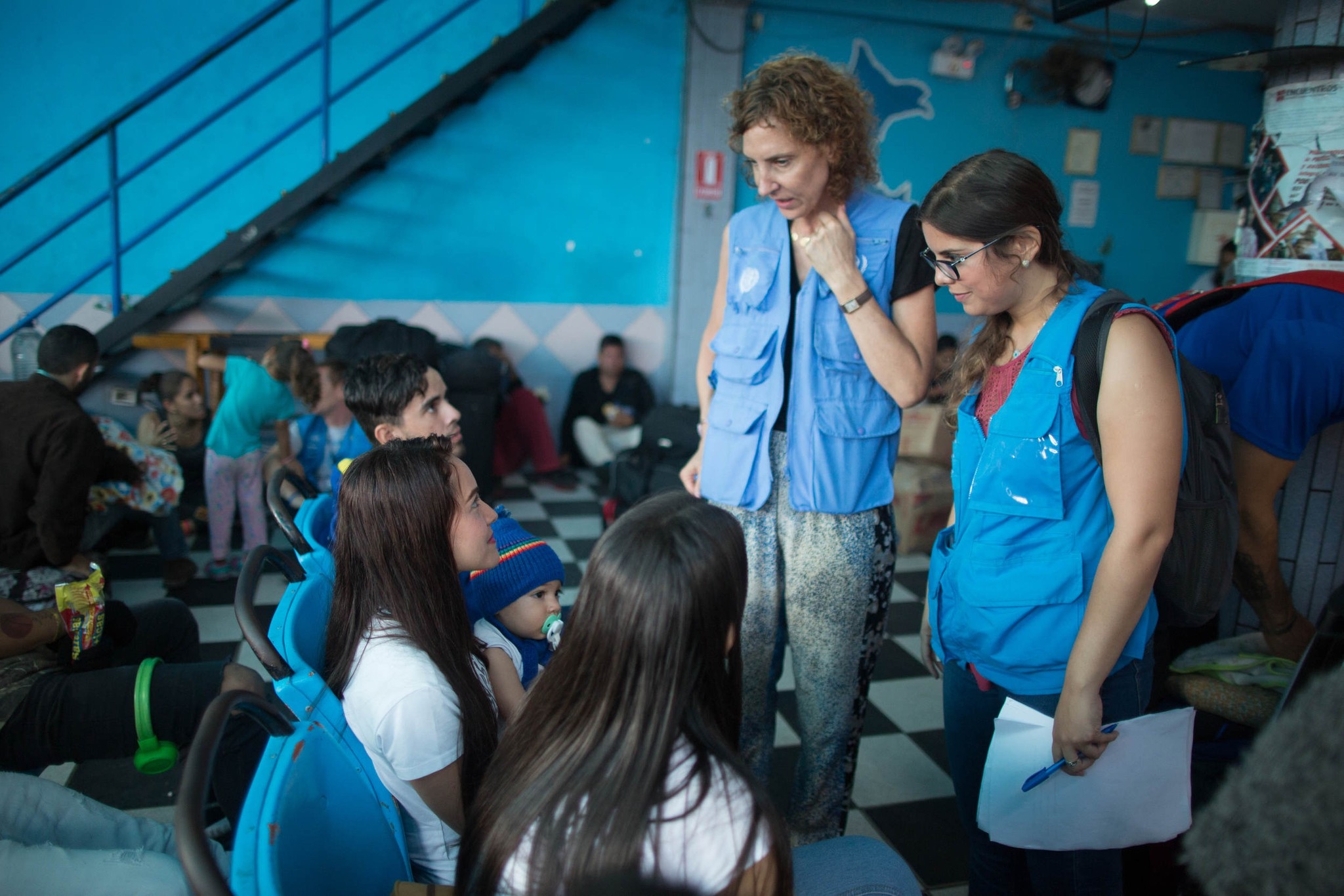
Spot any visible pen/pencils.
[1021,722,1118,793]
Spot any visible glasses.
[87,360,104,373]
[921,222,1032,279]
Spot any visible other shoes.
[165,559,195,589]
[204,559,229,581]
[230,558,248,577]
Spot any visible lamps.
[1029,40,1115,111]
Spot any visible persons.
[464,505,568,722]
[322,432,499,886]
[912,148,1188,896]
[200,317,562,583]
[453,495,777,896]
[136,368,218,505]
[559,333,657,483]
[678,49,939,846]
[1155,269,1343,758]
[0,596,271,845]
[0,771,236,896]
[1191,238,1239,292]
[0,325,200,590]
[928,333,958,401]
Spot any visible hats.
[470,504,565,619]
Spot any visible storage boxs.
[892,403,957,555]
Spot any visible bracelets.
[840,288,872,314]
[44,606,61,645]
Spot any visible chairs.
[176,467,412,896]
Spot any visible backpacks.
[1072,289,1239,625]
